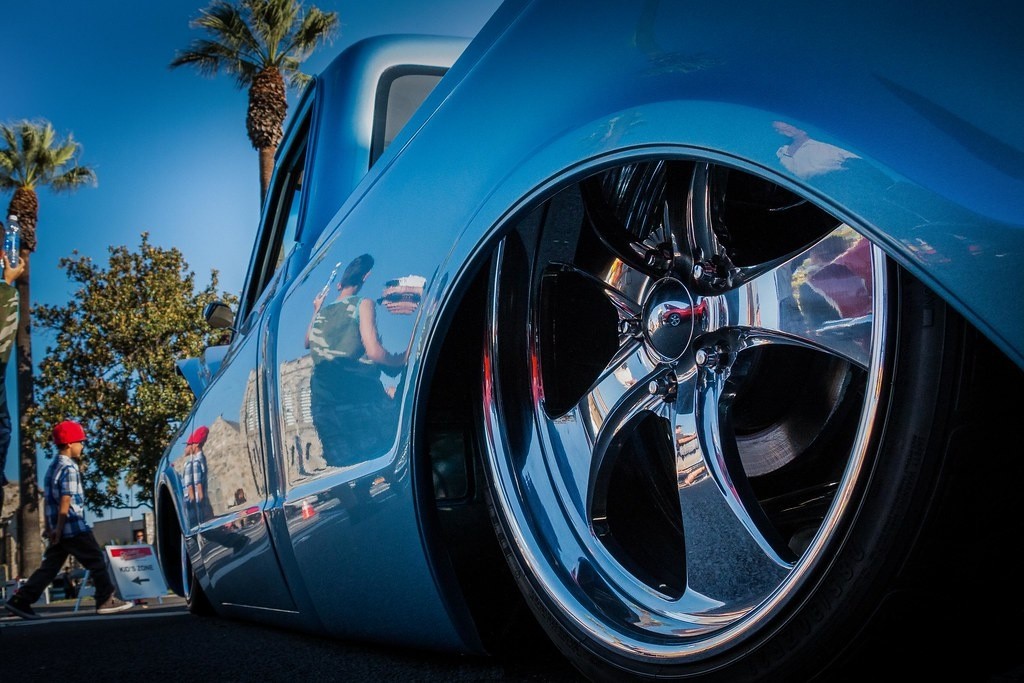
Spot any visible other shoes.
[319,481,407,516]
[348,502,371,523]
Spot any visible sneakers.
[96,595,133,614]
[4,595,42,620]
[230,535,251,560]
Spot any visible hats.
[386,274,426,287]
[52,421,87,445]
[181,426,209,445]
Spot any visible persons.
[64,567,77,598]
[380,275,425,315]
[304,254,405,516]
[4,421,135,619]
[132,530,148,605]
[183,427,251,557]
[0,220,25,516]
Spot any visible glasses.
[184,443,190,447]
[76,441,87,445]
[376,293,401,303]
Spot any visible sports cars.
[153,0,1024,683]
